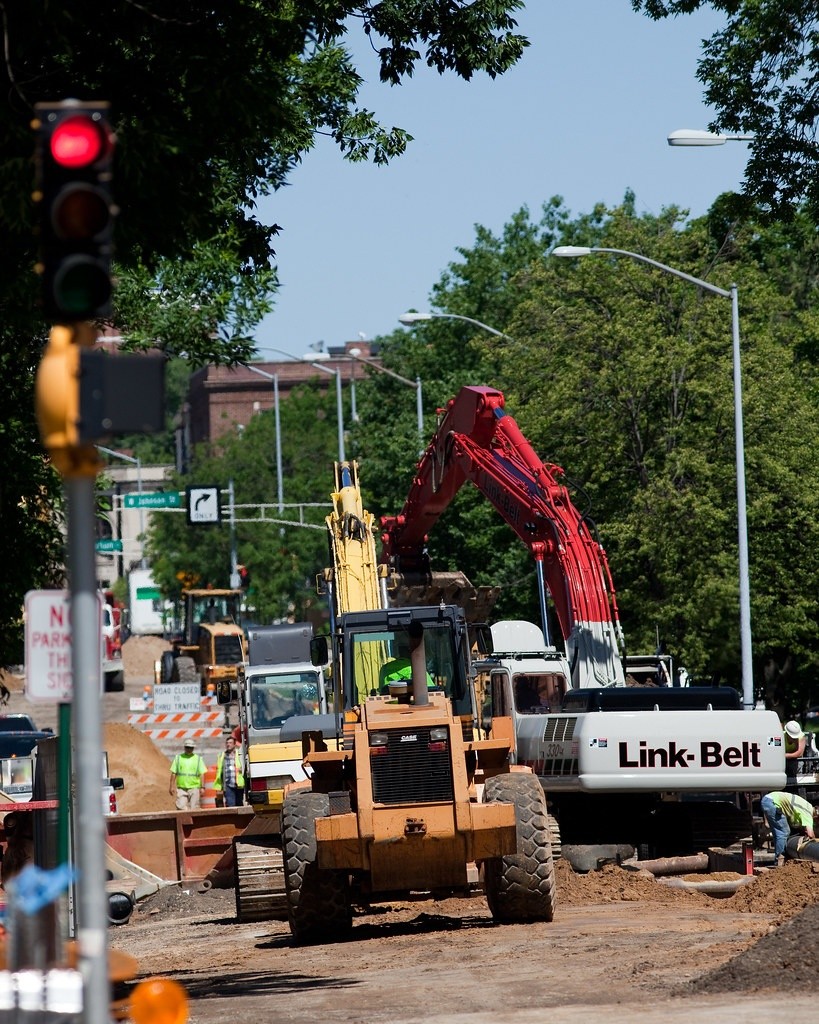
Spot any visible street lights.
[235,343,347,473]
[178,351,286,539]
[552,243,753,708]
[300,349,426,457]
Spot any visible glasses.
[185,747,193,749]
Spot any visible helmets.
[397,632,410,646]
[784,720,804,739]
[182,739,197,748]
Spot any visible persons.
[379,633,436,696]
[168,739,208,810]
[784,733,806,758]
[211,735,245,807]
[761,791,819,866]
[206,598,219,622]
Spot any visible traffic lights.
[28,100,119,318]
[31,326,167,478]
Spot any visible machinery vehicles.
[231,599,559,943]
[372,378,787,868]
[152,582,251,696]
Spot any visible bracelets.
[201,786,205,789]
